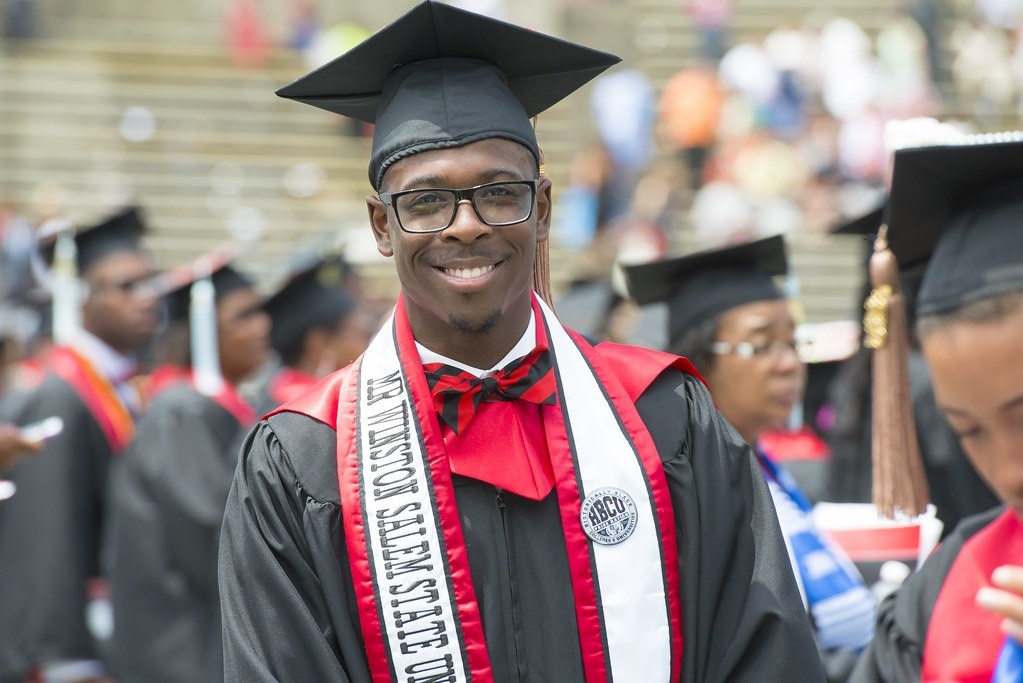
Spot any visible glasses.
[708,337,812,362]
[378,178,542,234]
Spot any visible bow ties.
[424,345,560,435]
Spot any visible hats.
[621,234,789,341]
[133,238,245,395]
[275,0,624,321]
[863,140,1023,518]
[37,208,147,346]
[236,242,361,347]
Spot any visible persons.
[854,141,1022,683]
[627,234,875,683]
[838,213,1004,539]
[213,1,833,683]
[0,206,379,683]
